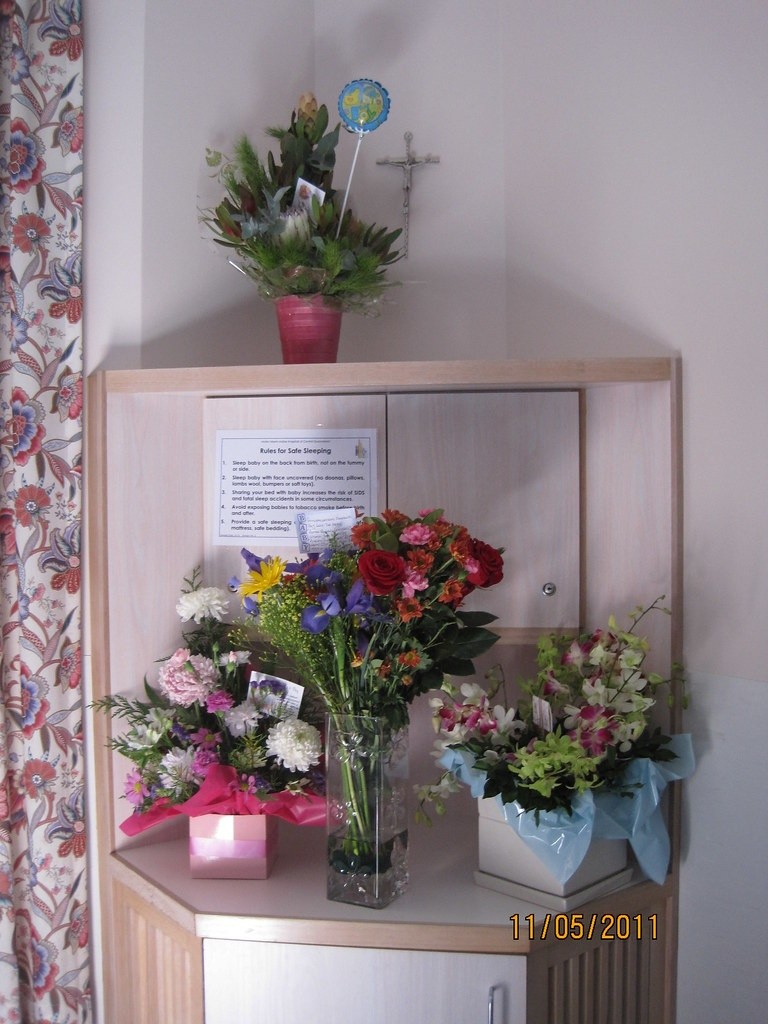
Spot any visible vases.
[184,816,271,881]
[322,716,413,903]
[277,300,342,361]
[471,790,634,912]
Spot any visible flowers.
[421,600,679,803]
[207,89,403,298]
[225,504,505,855]
[86,575,326,823]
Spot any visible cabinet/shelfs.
[89,352,683,1024]
